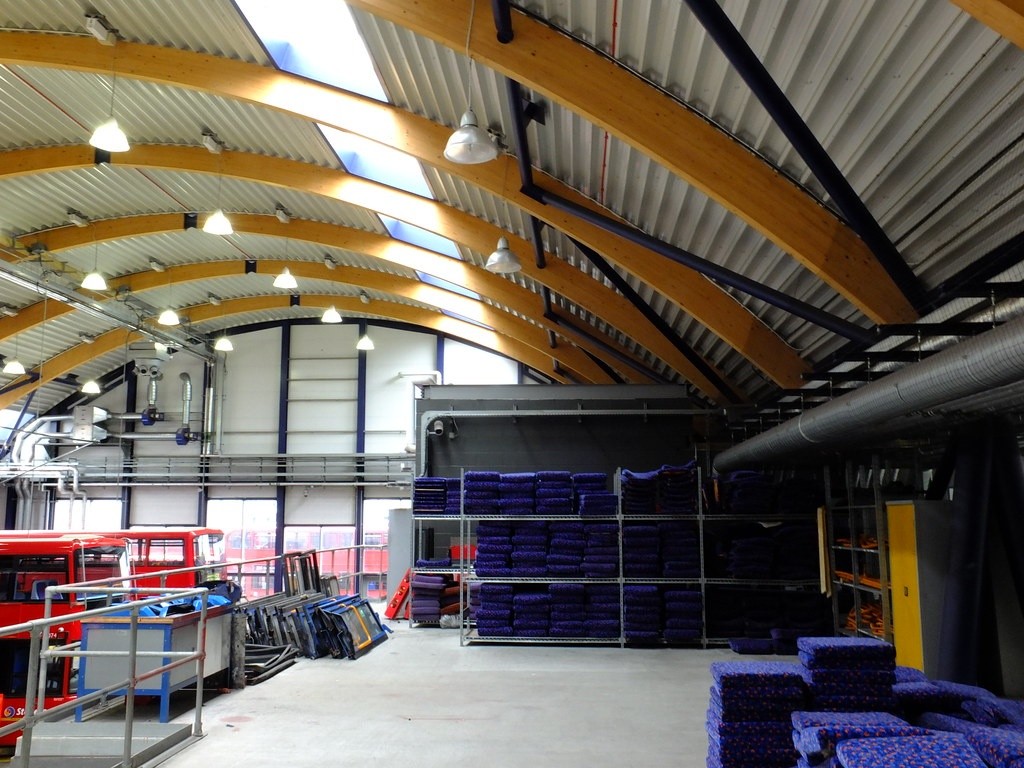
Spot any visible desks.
[74,601,237,723]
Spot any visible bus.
[0,527,228,602]
[0,533,135,760]
[218,528,388,603]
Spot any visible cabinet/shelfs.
[407,452,980,674]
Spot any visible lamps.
[214,314,233,351]
[80,241,108,290]
[273,239,298,288]
[87,74,131,153]
[81,356,100,394]
[201,175,233,236]
[2,332,27,375]
[356,317,374,351]
[484,200,522,275]
[443,56,498,164]
[321,279,342,324]
[158,283,180,325]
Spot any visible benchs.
[31,579,64,600]
